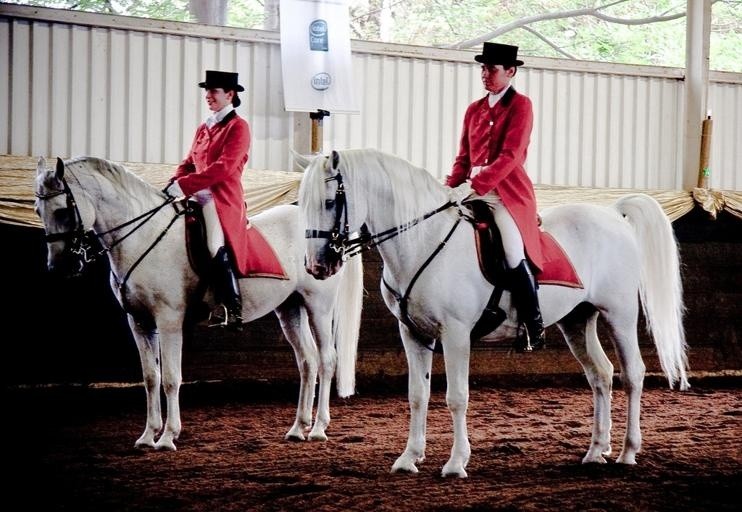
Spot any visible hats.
[474,42,524,66]
[198,71,244,92]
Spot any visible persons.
[444,42,549,353]
[166,71,252,335]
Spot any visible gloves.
[448,182,475,208]
[166,180,184,199]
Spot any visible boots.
[210,245,244,334]
[508,259,547,350]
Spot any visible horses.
[288,145,693,481]
[33,155,364,453]
